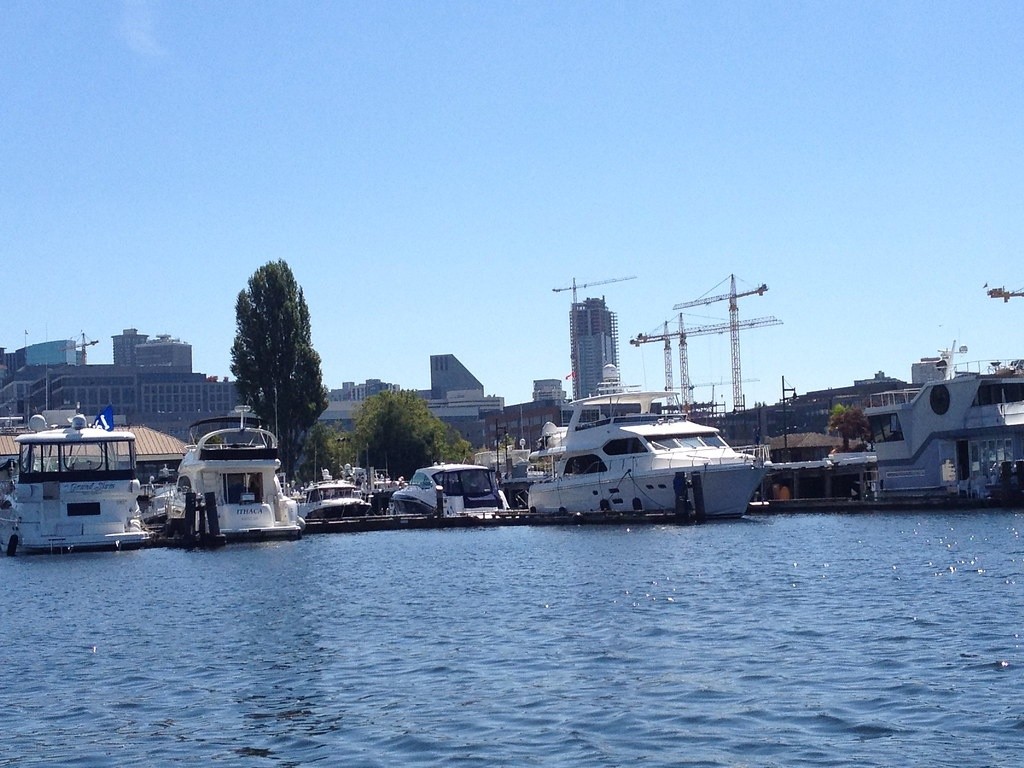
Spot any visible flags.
[95,403,114,432]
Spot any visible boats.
[524,364,776,521]
[0,414,153,558]
[139,460,510,524]
[166,404,305,544]
[861,340,1024,513]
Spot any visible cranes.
[982,281,1024,303]
[552,276,637,399]
[629,274,784,447]
[59,332,100,365]
